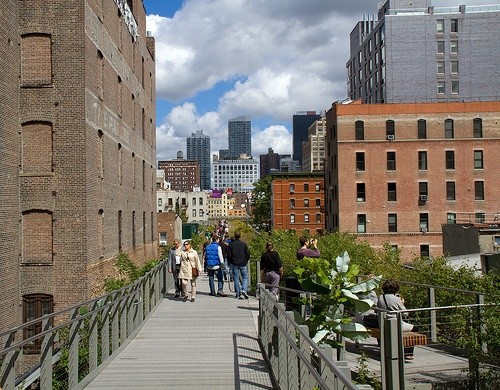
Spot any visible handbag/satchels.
[192,268,198,277]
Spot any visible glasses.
[184,244,189,245]
[174,244,178,245]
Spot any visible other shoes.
[217,290,227,297]
[183,296,188,302]
[236,294,243,299]
[192,298,195,302]
[406,355,413,360]
[209,291,215,296]
[242,291,249,300]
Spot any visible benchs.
[371,328,426,360]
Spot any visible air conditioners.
[422,228,427,232]
[388,135,394,139]
[420,195,428,201]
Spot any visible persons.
[260,241,283,295]
[354,274,380,347]
[202,219,236,282]
[377,280,418,362]
[175,240,202,302]
[256,225,259,230]
[205,236,227,297]
[296,236,320,318]
[227,232,250,299]
[168,239,185,298]
